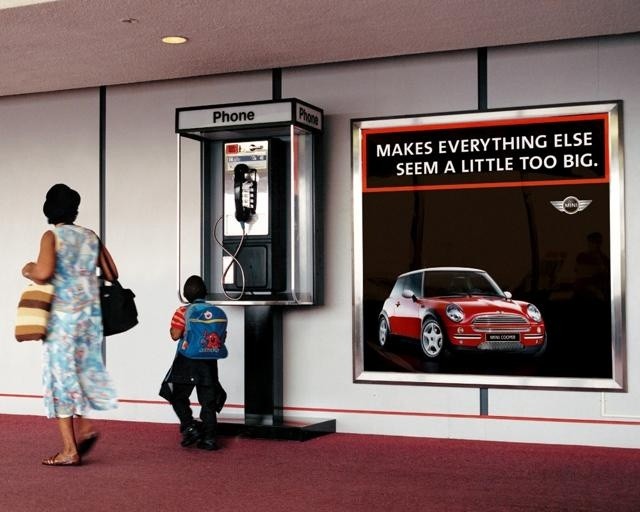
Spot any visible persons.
[157,275,228,448]
[20,183,137,466]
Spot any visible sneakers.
[180,420,221,451]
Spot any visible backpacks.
[176,297,229,359]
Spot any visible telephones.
[234,164,251,222]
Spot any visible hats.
[43,184,80,216]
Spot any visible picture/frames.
[349,99,632,396]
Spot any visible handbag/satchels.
[93,236,140,337]
[14,279,56,343]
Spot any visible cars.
[378,266,548,360]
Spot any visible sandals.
[42,450,82,467]
